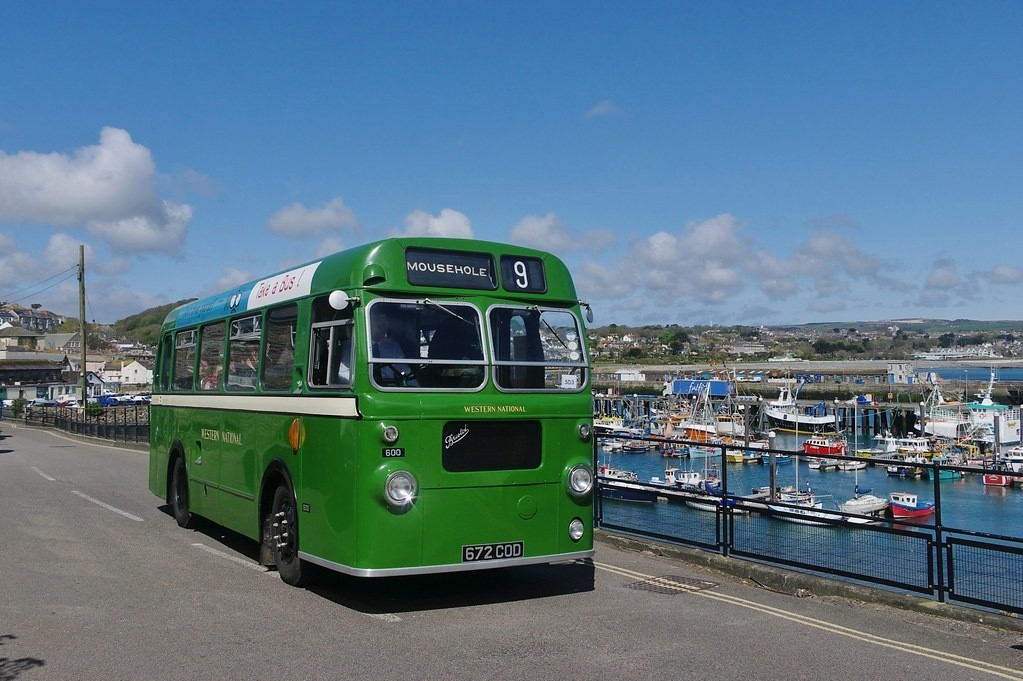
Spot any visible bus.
[148,237,599,589]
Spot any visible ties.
[373,343,382,383]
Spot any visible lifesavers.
[836,442,842,450]
[979,413,985,420]
[672,450,675,454]
[710,447,715,452]
[664,450,668,455]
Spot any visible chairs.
[427,324,482,370]
[176,376,193,390]
[217,370,240,391]
[265,361,285,389]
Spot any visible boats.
[594,356,1023,529]
[889,490,936,520]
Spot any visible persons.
[338,311,421,388]
[199,346,256,390]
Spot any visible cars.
[0,388,152,407]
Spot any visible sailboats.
[836,398,889,518]
